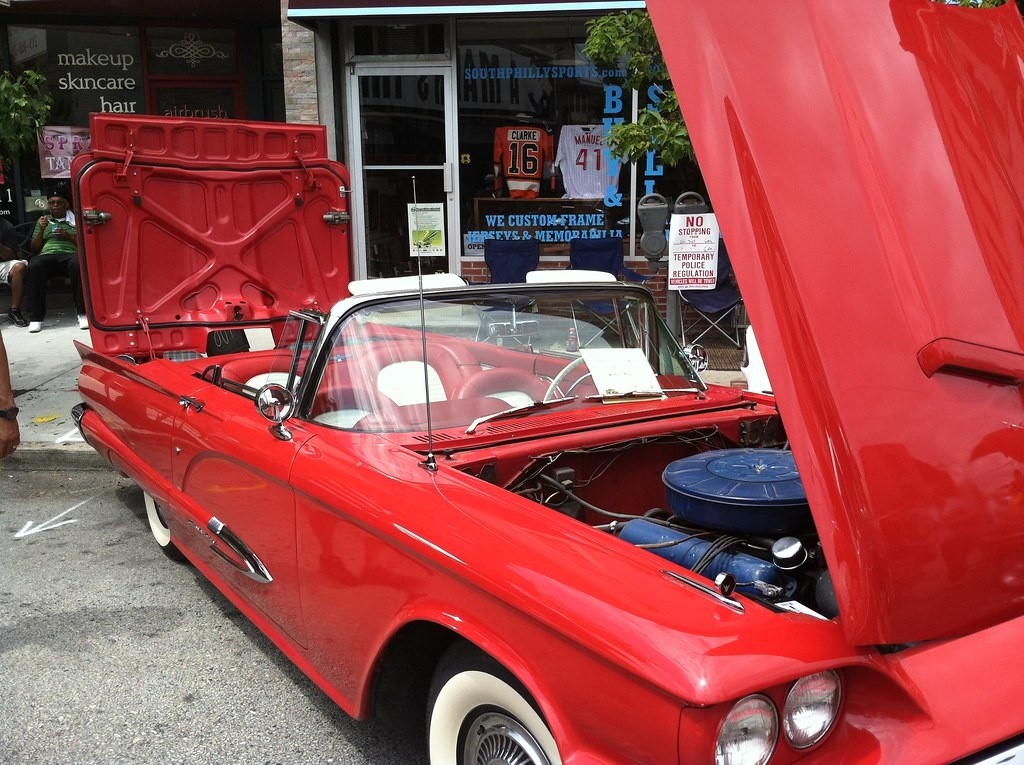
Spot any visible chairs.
[666,241,745,352]
[219,353,330,411]
[308,388,396,436]
[472,238,542,349]
[364,342,467,405]
[565,236,653,348]
[452,367,558,415]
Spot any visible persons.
[0,201,30,328]
[25,189,89,333]
[0,335,23,460]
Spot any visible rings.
[12,445,17,450]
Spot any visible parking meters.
[638,191,710,342]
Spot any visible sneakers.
[29,322,43,332]
[8,306,27,326]
[77,314,89,329]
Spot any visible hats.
[47,189,66,200]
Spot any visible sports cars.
[67,0,1024,765]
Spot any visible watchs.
[0,406,20,419]
